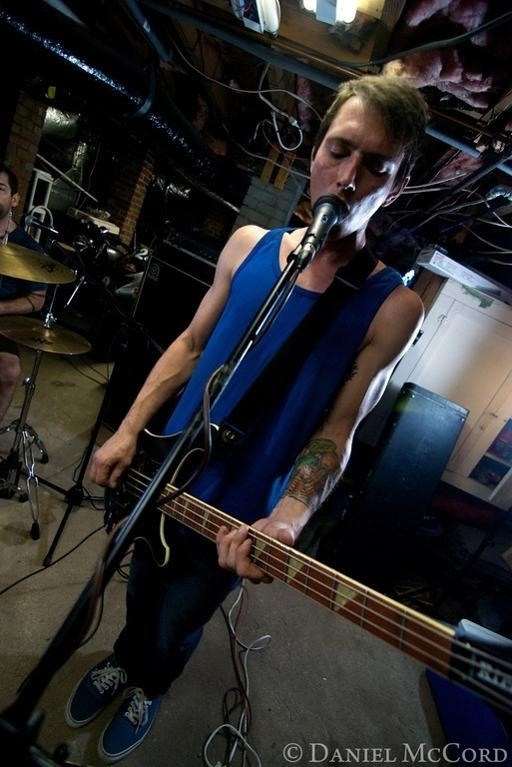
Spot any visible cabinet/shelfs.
[354,264,511,512]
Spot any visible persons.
[0,161,52,424]
[61,73,425,760]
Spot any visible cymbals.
[0,315,91,355]
[0,239,75,284]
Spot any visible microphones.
[22,213,62,236]
[294,190,349,276]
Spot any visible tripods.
[0,357,50,540]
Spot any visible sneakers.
[63,652,134,728]
[97,687,163,765]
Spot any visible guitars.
[103,423,512,714]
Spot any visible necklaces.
[4,218,10,237]
